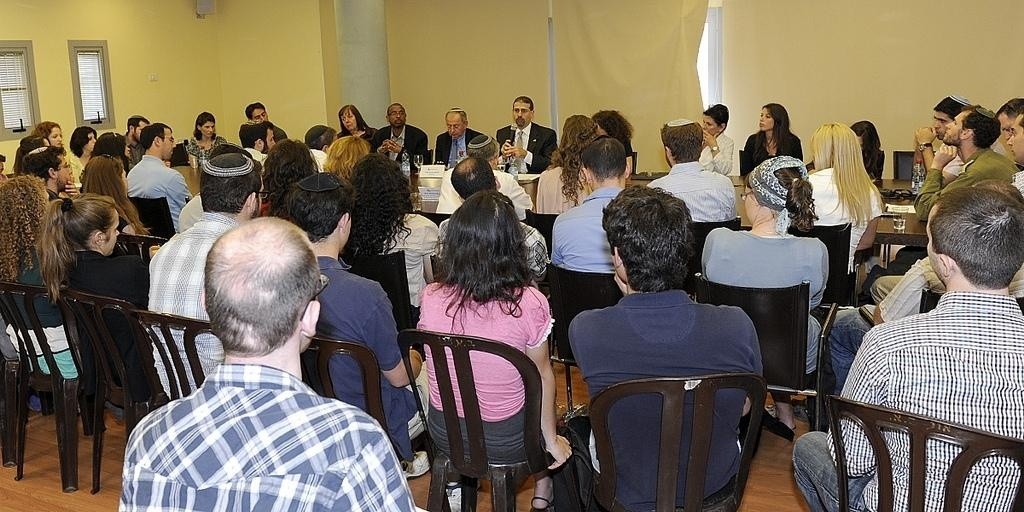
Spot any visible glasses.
[58,159,72,168]
[244,190,270,205]
[741,191,754,201]
[300,272,329,317]
[102,153,113,161]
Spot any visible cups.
[892,207,909,231]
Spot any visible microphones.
[509,123,518,146]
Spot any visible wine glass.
[497,161,505,172]
[414,155,423,174]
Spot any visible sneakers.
[400,451,431,480]
[791,396,828,431]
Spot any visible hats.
[468,134,492,148]
[450,107,463,112]
[129,115,144,119]
[28,146,48,155]
[297,171,341,192]
[305,125,328,146]
[666,118,696,127]
[202,152,255,178]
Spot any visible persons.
[1,95,1024,512]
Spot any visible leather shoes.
[766,403,797,430]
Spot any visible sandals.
[529,495,554,512]
[446,479,481,496]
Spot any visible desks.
[170,163,207,196]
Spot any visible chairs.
[881,151,914,264]
[632,152,638,173]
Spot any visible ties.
[514,132,524,173]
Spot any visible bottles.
[508,161,518,183]
[912,159,926,195]
[401,149,410,176]
